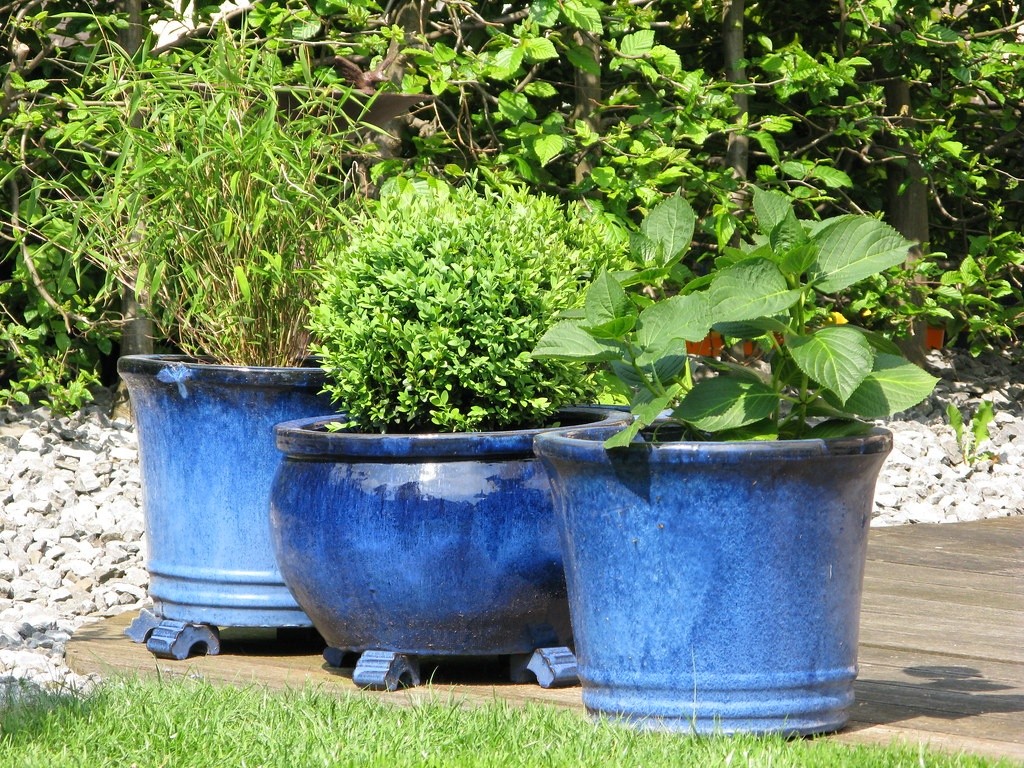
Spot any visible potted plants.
[58,55,342,665]
[269,168,623,685]
[528,175,943,740]
